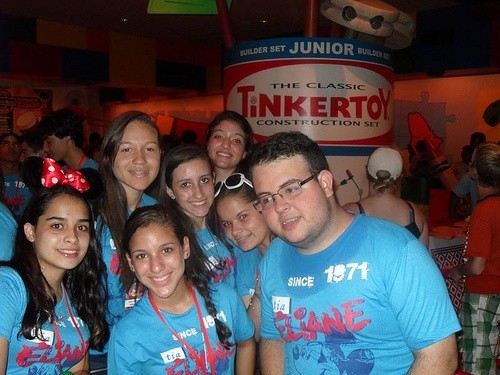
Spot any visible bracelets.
[249,293,261,314]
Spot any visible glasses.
[251,172,318,213]
[214,173,253,197]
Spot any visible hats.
[368,147,403,181]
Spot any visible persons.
[340,146,430,252]
[458,143,500,375]
[156,144,265,341]
[92,109,163,331]
[249,131,463,375]
[204,110,254,191]
[403,131,487,218]
[208,172,275,254]
[107,204,256,375]
[0,158,108,374]
[0,107,104,263]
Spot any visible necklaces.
[195,228,226,267]
